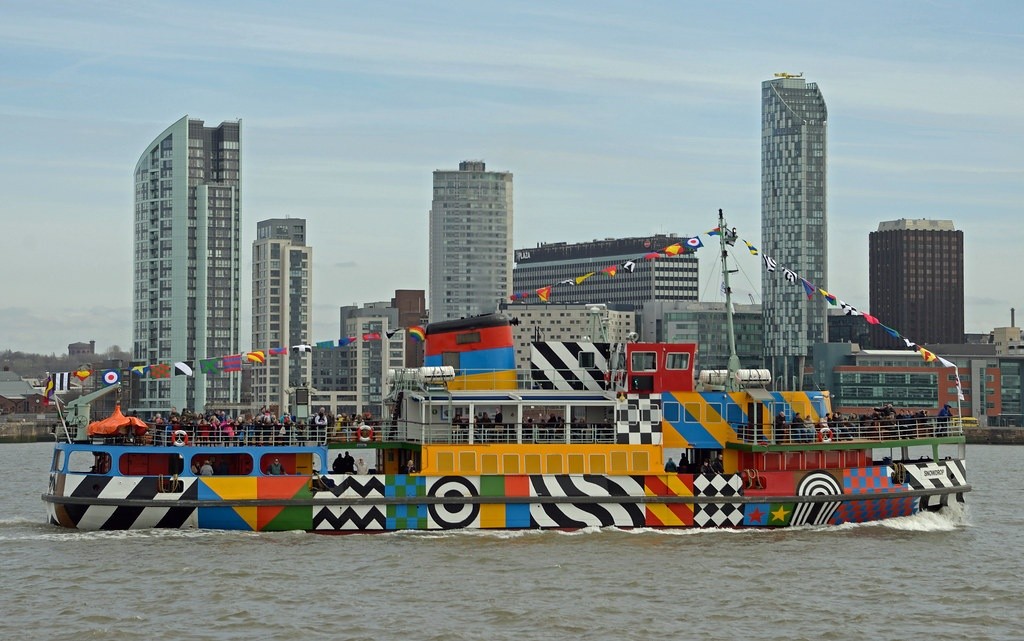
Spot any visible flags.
[725,229,939,362]
[45,325,425,401]
[509,230,723,300]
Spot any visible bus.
[952,416,979,427]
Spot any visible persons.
[354,458,369,475]
[404,461,416,474]
[664,453,686,473]
[193,458,229,476]
[775,412,786,443]
[571,418,588,439]
[476,407,503,442]
[525,413,564,439]
[601,417,613,438]
[936,404,953,436]
[452,415,462,426]
[332,451,354,474]
[267,458,288,475]
[792,404,928,443]
[701,454,722,474]
[148,405,376,446]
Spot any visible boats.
[40,207,972,538]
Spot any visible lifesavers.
[171,430,189,447]
[819,428,833,443]
[357,425,373,442]
